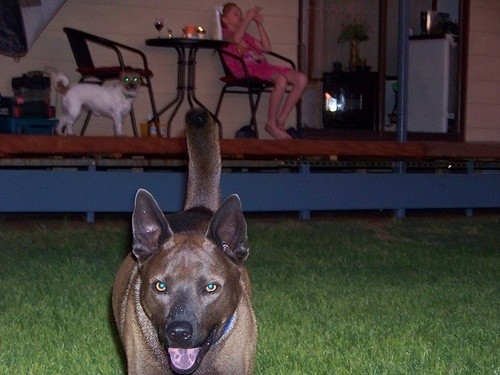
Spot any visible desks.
[145,38,230,140]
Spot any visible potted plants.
[337,24,370,66]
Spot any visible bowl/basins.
[141,123,168,137]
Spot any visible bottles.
[420,9,438,36]
[197,29,206,40]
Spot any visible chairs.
[213,46,303,138]
[63,26,161,138]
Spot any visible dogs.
[41,64,155,135]
[111,105,257,375]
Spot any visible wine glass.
[154,17,165,39]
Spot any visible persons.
[221,2,308,139]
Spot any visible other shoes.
[236,126,256,137]
[286,128,300,137]
